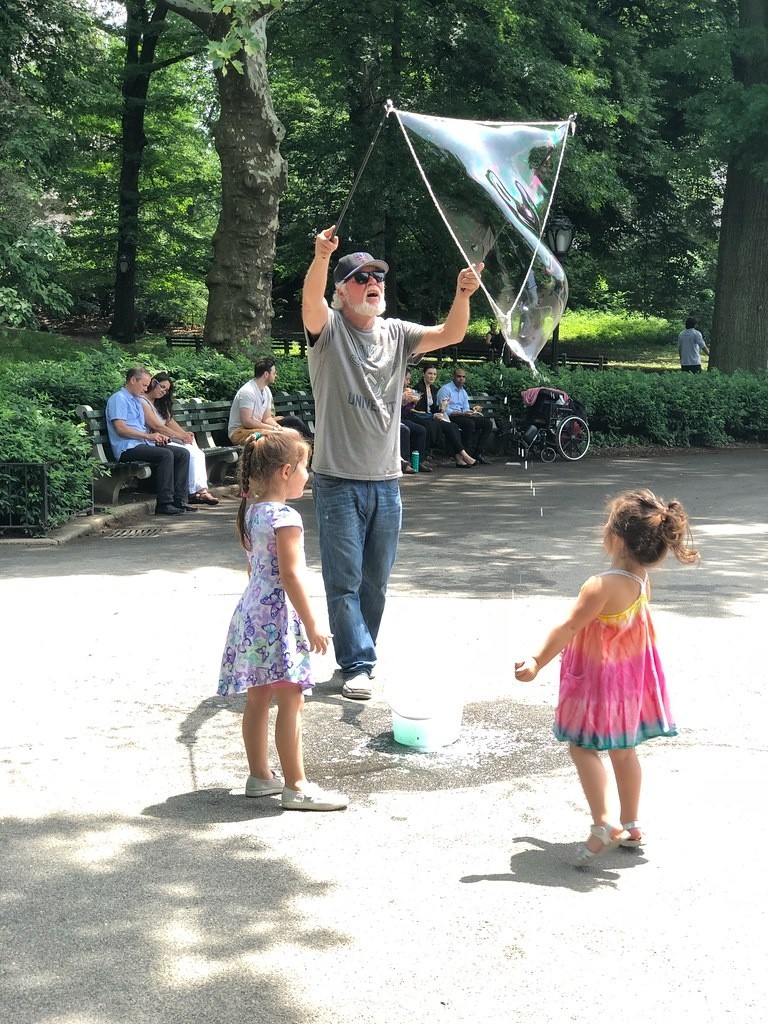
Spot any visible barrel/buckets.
[385,667,466,749]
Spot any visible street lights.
[546,205,573,376]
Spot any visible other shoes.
[343,671,371,699]
[174,501,199,513]
[477,456,493,464]
[420,464,434,472]
[456,463,472,468]
[188,494,219,505]
[155,504,186,515]
[465,459,482,466]
[403,465,418,474]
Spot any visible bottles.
[411,451,420,473]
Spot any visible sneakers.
[245,770,285,796]
[282,783,350,810]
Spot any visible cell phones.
[156,439,173,446]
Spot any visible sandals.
[572,820,631,868]
[620,821,644,847]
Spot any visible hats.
[333,252,389,285]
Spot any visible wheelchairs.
[508,384,591,463]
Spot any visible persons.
[107,367,198,513]
[678,317,709,373]
[216,430,350,810]
[412,365,480,467]
[226,363,283,445]
[400,423,417,473]
[485,324,518,365]
[141,374,218,505]
[272,399,314,453]
[401,368,434,471]
[302,226,484,699]
[514,487,702,866]
[437,369,492,464]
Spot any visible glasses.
[157,381,169,395]
[345,271,386,284]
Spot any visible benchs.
[75,391,502,504]
[166,334,608,372]
